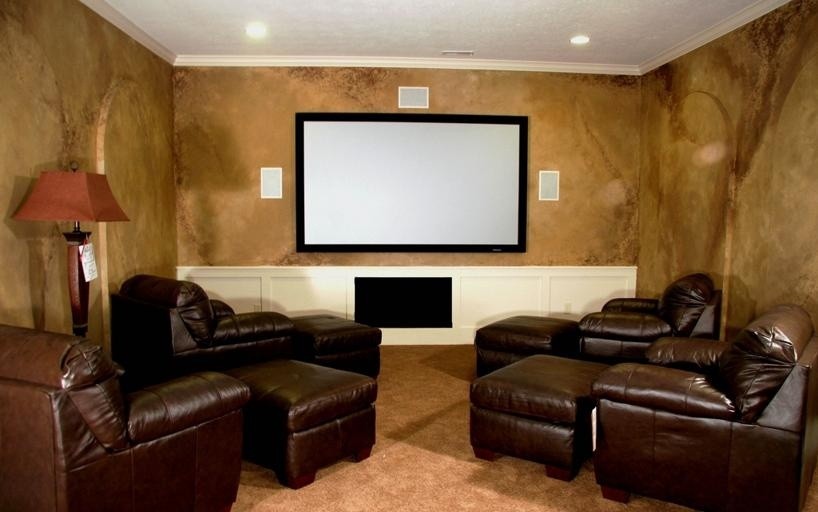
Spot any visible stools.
[224,358,378,489]
[475,315,580,378]
[289,314,382,379]
[470,354,613,483]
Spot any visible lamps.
[11,170,132,337]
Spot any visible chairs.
[577,273,722,366]
[109,273,294,385]
[591,304,818,512]
[0,324,251,511]
